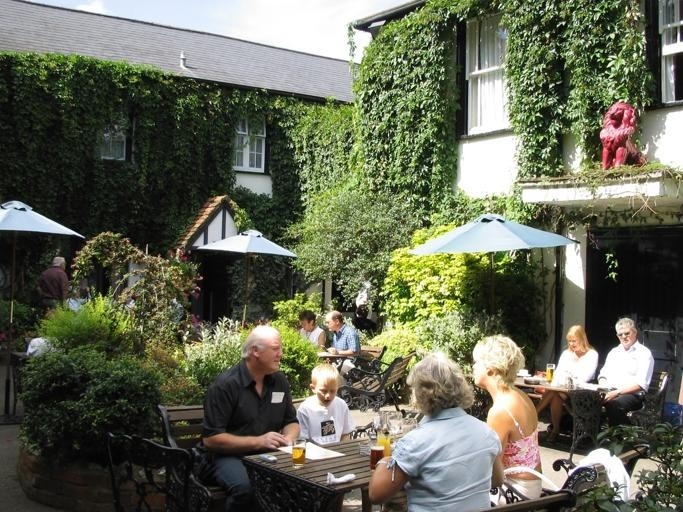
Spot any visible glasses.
[617,332,631,339]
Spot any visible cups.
[377,434,390,457]
[401,419,418,437]
[545,363,554,382]
[370,445,384,473]
[292,438,306,469]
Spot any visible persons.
[36,256,69,312]
[535,324,599,445]
[203,323,300,512]
[298,309,327,348]
[323,310,361,381]
[598,317,654,439]
[296,363,353,512]
[368,351,503,512]
[470,333,542,509]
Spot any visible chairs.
[348,346,387,372]
[552,443,651,495]
[587,363,673,429]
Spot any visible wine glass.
[386,413,402,446]
[373,412,385,435]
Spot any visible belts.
[42,296,57,300]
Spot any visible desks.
[513,376,617,461]
[240,431,405,512]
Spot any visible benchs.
[336,349,417,415]
[104,431,211,512]
[156,398,310,511]
[469,488,577,512]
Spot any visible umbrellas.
[198,229,297,328]
[0,199,85,369]
[408,213,582,320]
